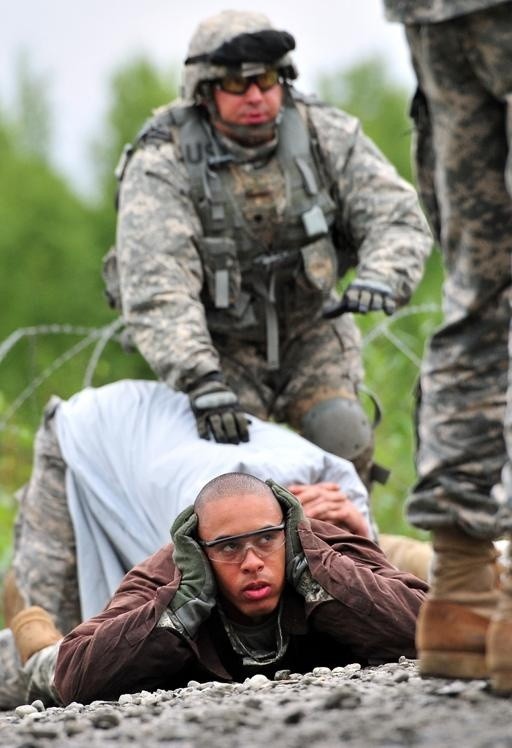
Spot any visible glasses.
[197,525,287,565]
[211,66,279,95]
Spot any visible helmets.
[177,9,295,108]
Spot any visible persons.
[9,469,427,710]
[381,0,512,698]
[97,6,436,498]
[2,378,377,712]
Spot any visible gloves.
[263,478,308,590]
[189,381,251,445]
[320,281,396,320]
[168,504,216,640]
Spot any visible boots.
[376,532,511,698]
[3,570,62,668]
